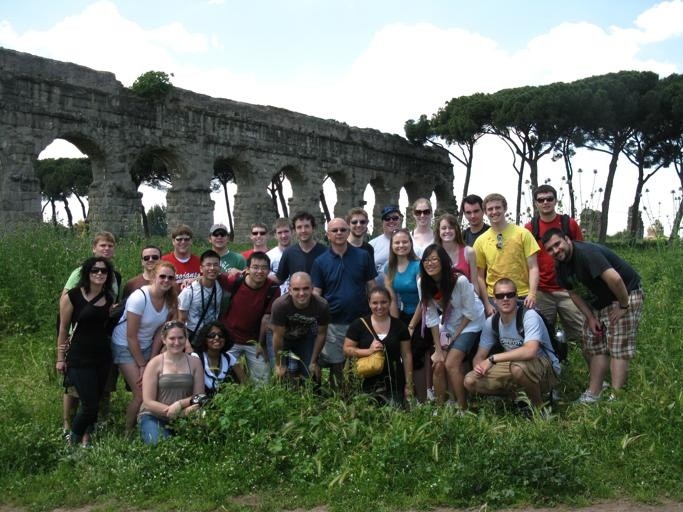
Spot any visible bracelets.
[136,363,148,368]
[176,399,183,411]
[55,359,65,363]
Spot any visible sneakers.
[427,387,561,424]
[62,432,73,454]
[574,382,624,403]
[80,440,95,450]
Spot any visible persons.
[540,227,645,405]
[55,230,128,441]
[108,261,184,439]
[120,244,162,301]
[135,322,209,446]
[51,255,123,453]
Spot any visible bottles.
[553,321,566,343]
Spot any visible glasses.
[141,254,160,262]
[200,262,222,270]
[535,196,555,203]
[203,331,225,339]
[248,264,269,271]
[492,290,517,300]
[382,215,401,221]
[496,233,502,250]
[421,257,440,264]
[89,266,109,275]
[160,320,186,333]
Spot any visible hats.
[209,223,229,233]
[380,206,404,220]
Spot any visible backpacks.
[492,307,559,364]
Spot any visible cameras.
[553,329,568,344]
[190,393,209,408]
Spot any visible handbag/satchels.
[434,332,449,349]
[64,351,74,389]
[350,350,387,376]
